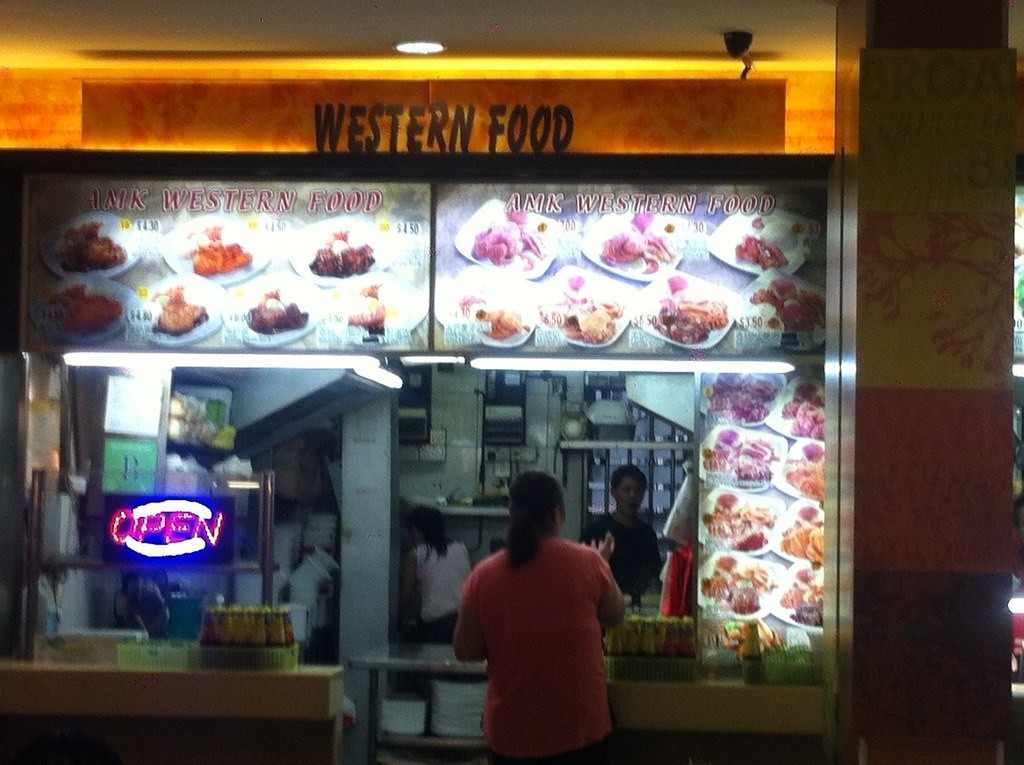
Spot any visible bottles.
[606,612,698,681]
[198,605,295,669]
[741,623,761,682]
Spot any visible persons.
[454,472,626,765]
[399,507,472,645]
[574,464,661,611]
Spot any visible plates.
[732,268,824,349]
[634,271,737,350]
[30,278,134,344]
[699,487,784,556]
[44,211,143,279]
[329,274,428,336]
[699,425,788,492]
[700,375,787,426]
[766,563,825,633]
[764,375,799,440]
[707,204,820,276]
[128,273,230,345]
[436,295,536,346]
[287,217,395,286]
[221,274,320,346]
[697,549,787,621]
[454,199,563,279]
[537,265,636,346]
[773,438,826,502]
[580,213,683,282]
[157,215,270,284]
[770,499,824,563]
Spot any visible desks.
[348,643,491,765]
